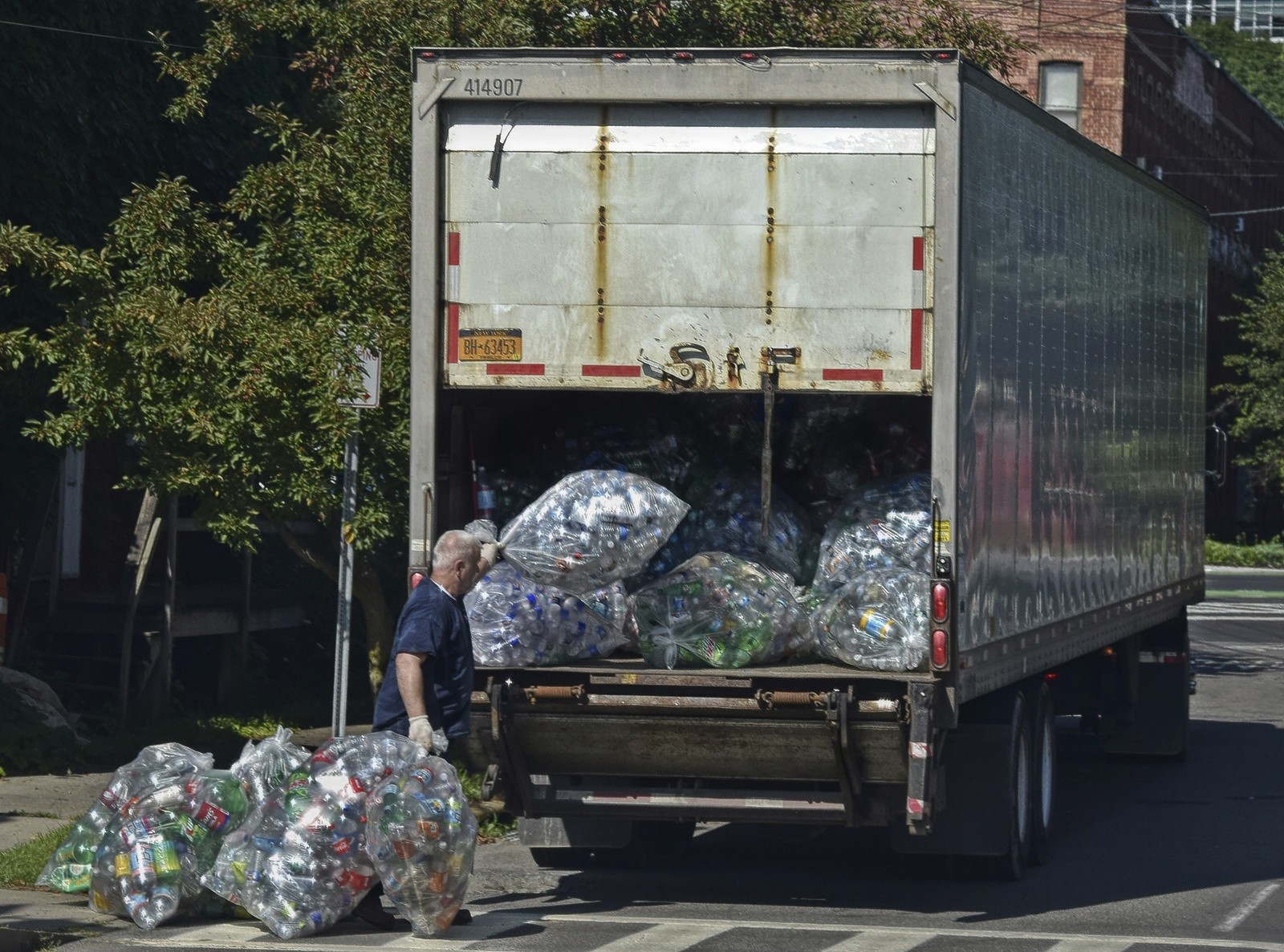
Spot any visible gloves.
[480,542,500,568]
[407,715,434,750]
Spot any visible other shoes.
[451,908,472,926]
[352,893,395,931]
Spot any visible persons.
[350,527,507,932]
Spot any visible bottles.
[200,730,427,940]
[41,741,216,891]
[476,407,809,594]
[365,754,477,937]
[623,552,813,670]
[783,395,931,530]
[809,472,930,593]
[91,770,255,932]
[809,567,931,673]
[462,560,627,666]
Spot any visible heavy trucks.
[407,40,1216,881]
